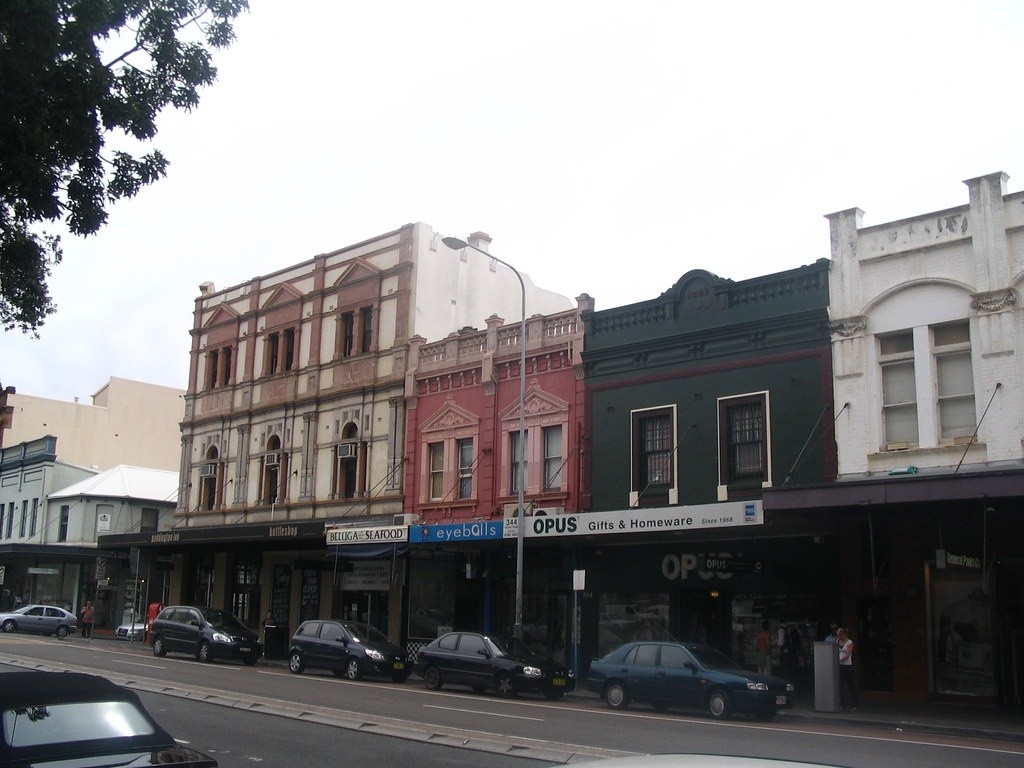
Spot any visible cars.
[146,605,264,666]
[586,641,796,720]
[414,631,576,701]
[114,616,150,641]
[0,662,220,768]
[0,604,77,637]
[288,620,415,684]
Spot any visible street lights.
[442,237,525,641]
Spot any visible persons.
[261,610,276,645]
[754,621,858,710]
[80,600,95,638]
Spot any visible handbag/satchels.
[80,607,86,619]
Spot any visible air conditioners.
[264,453,279,466]
[392,514,420,525]
[200,464,217,479]
[504,503,532,518]
[337,444,357,459]
[532,507,565,516]
